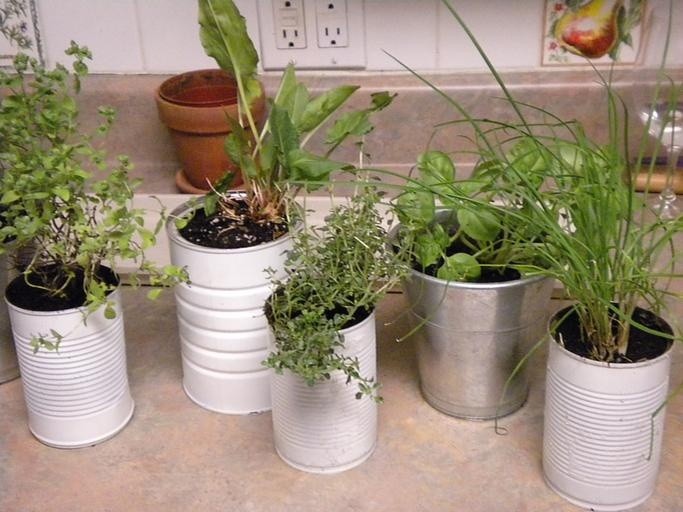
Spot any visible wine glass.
[630,59,683,221]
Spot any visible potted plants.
[0,1,138,449]
[250,122,406,478]
[403,0,673,509]
[154,6,394,417]
[387,132,588,424]
[158,68,273,201]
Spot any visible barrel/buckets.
[263,286,380,473]
[0,227,48,385]
[164,189,307,415]
[4,264,136,449]
[382,205,566,420]
[542,298,676,512]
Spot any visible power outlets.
[313,0,349,50]
[270,0,308,52]
